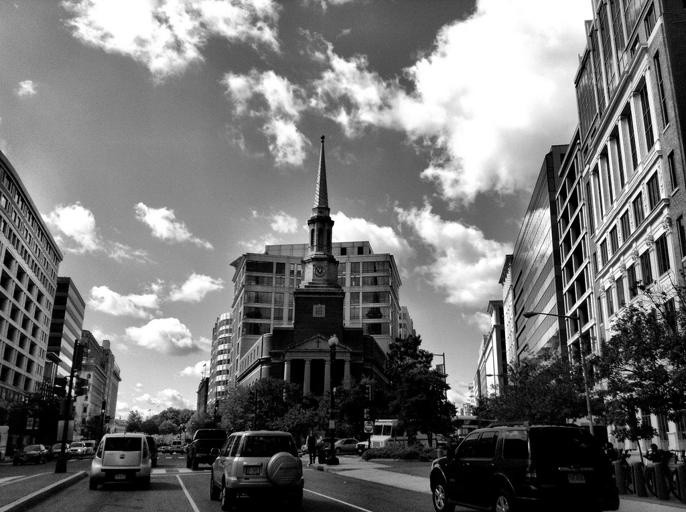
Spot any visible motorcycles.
[610,446,637,494]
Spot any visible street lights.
[525,311,596,439]
[327,334,339,464]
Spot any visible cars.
[299,435,342,457]
[22,438,95,465]
[334,438,360,455]
[146,435,158,467]
[89,430,153,489]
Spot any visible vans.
[157,439,184,454]
[356,417,437,456]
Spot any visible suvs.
[207,429,307,512]
[186,426,228,470]
[428,423,624,512]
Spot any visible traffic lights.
[74,342,84,369]
[51,376,66,397]
[75,376,88,395]
[101,400,106,414]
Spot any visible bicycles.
[645,449,686,502]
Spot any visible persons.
[604,441,621,476]
[304,429,317,464]
[315,435,326,463]
[643,443,677,489]
